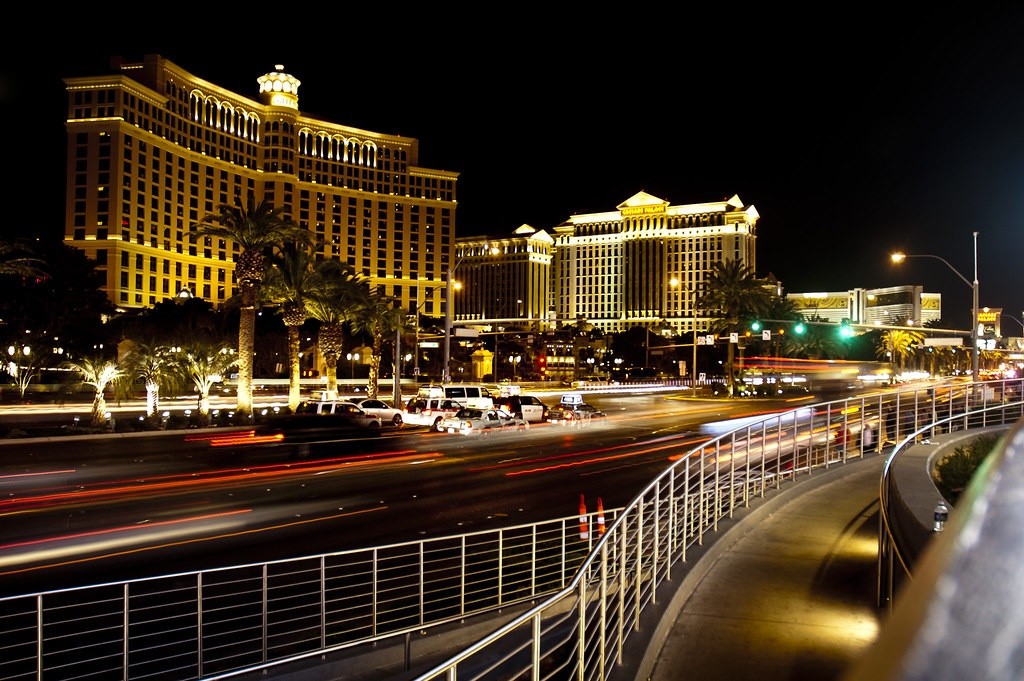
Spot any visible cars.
[295,384,552,433]
[543,395,608,429]
[440,407,529,433]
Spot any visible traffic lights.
[746,331,752,346]
[778,327,786,350]
[795,314,804,334]
[751,315,760,331]
[714,334,720,349]
[841,318,850,339]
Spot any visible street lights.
[403,353,411,378]
[586,358,595,375]
[891,253,978,408]
[494,297,522,383]
[414,283,462,383]
[509,356,521,376]
[7,345,31,402]
[347,353,359,378]
[671,279,697,398]
